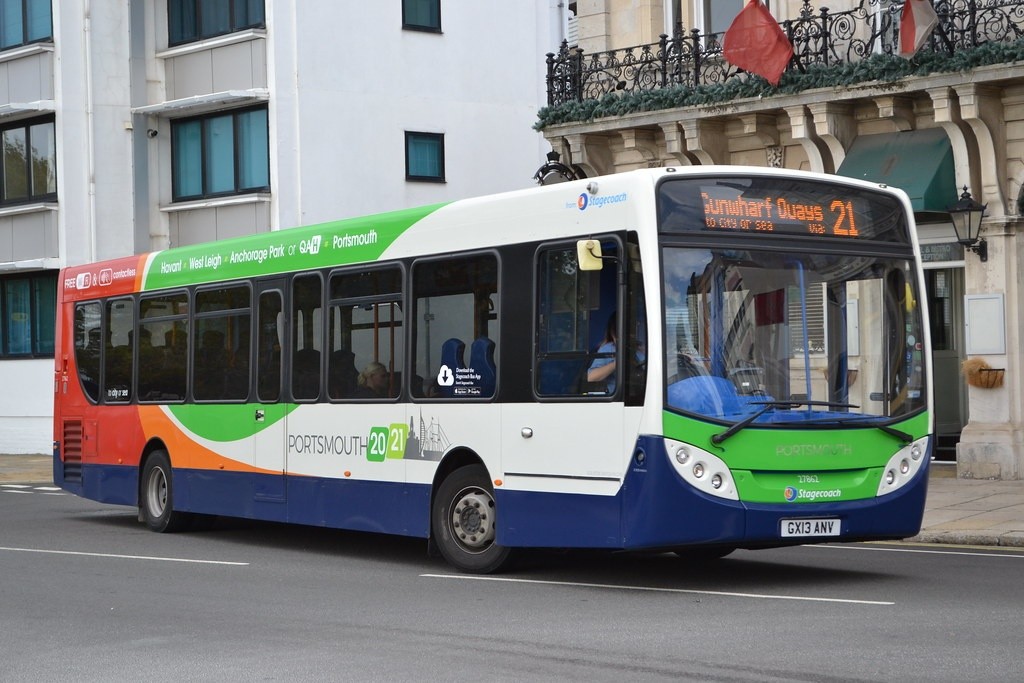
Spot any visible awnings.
[834,126,959,215]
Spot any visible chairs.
[72,326,495,400]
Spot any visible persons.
[354,361,390,399]
[585,309,645,395]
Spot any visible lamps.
[949,185,989,261]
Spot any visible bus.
[52,166,934,573]
